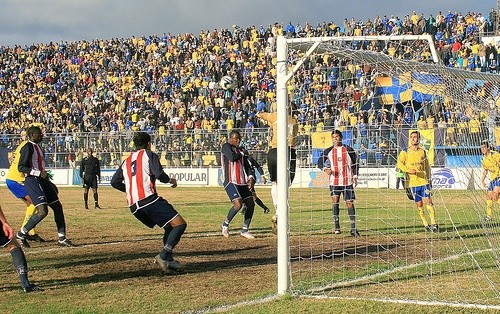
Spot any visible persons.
[239,146,270,214]
[256,99,298,234]
[14,126,78,247]
[219,130,256,240]
[479,140,500,221]
[0,7,500,168]
[0,205,40,293]
[80,148,102,210]
[318,130,360,238]
[110,132,188,274]
[396,131,439,232]
[5,123,46,242]
[395,165,406,190]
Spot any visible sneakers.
[221,223,229,238]
[154,255,172,273]
[26,233,45,242]
[167,261,184,269]
[241,232,255,238]
[335,230,340,234]
[14,231,30,249]
[350,229,360,237]
[271,215,277,235]
[57,239,77,247]
[23,283,37,293]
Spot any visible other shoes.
[85,206,89,209]
[239,211,244,215]
[426,225,431,231]
[432,223,439,232]
[264,208,270,214]
[95,205,101,210]
